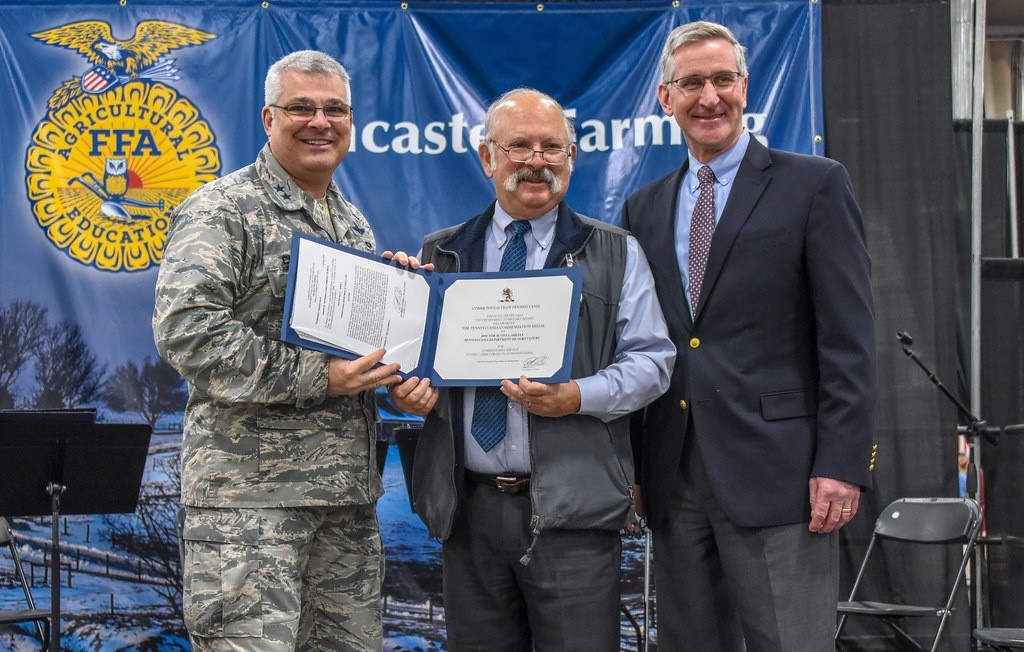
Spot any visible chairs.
[833,496,1024,652]
[0,516,53,652]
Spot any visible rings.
[528,402,531,409]
[842,509,851,513]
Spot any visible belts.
[463,467,532,495]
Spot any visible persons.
[622,21,876,652]
[387,87,677,652]
[151,49,436,652]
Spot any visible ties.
[470,219,531,453]
[689,165,716,320]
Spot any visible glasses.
[665,71,744,97]
[270,101,354,123]
[490,139,572,165]
